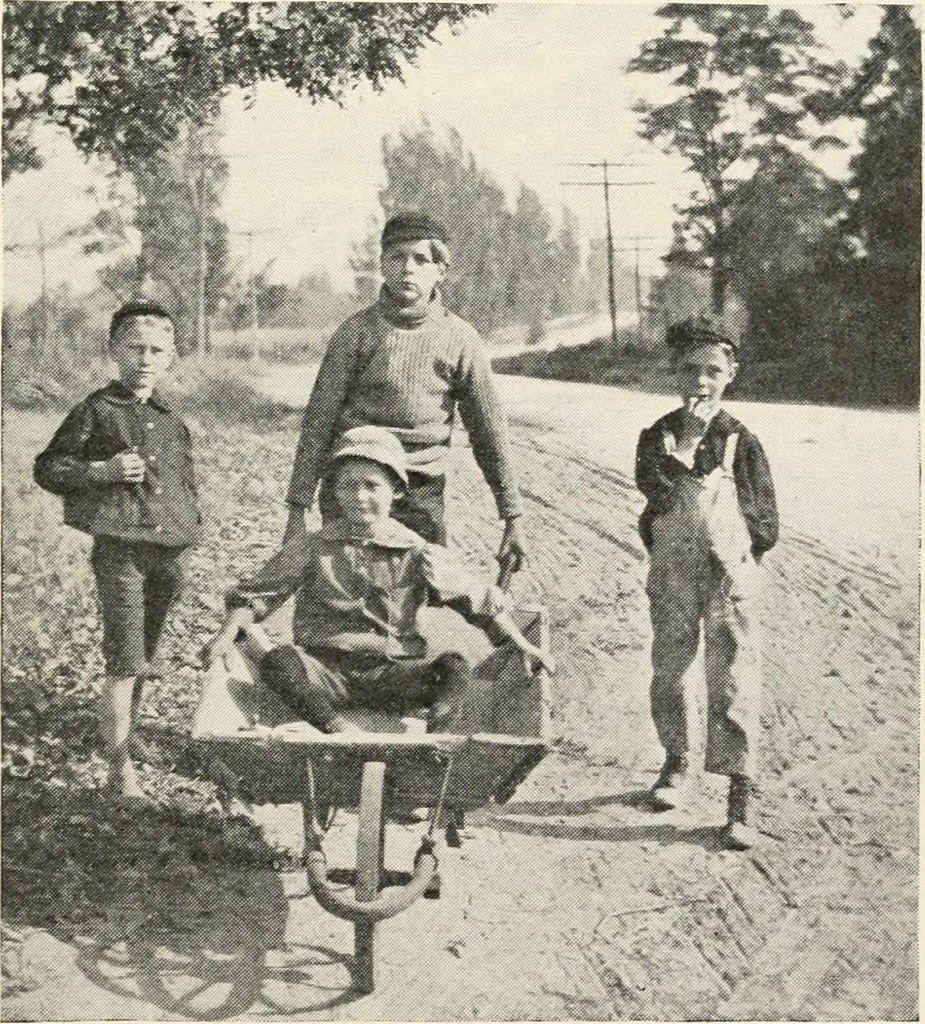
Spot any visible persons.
[194,422,557,735]
[633,310,780,851]
[30,294,206,801]
[278,211,534,571]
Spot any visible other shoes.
[132,736,162,763]
[106,779,153,813]
[648,760,688,808]
[725,783,758,846]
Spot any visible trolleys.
[187,547,553,995]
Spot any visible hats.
[381,210,448,251]
[664,317,741,352]
[109,299,176,341]
[329,425,409,485]
